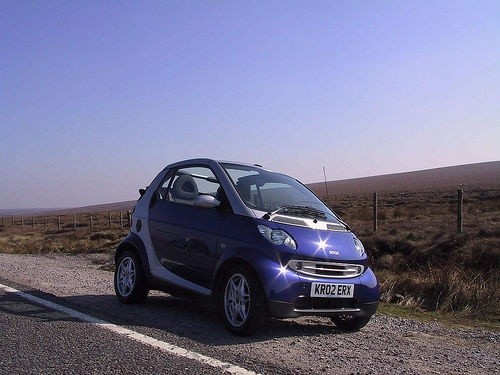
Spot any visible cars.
[114,158,381,338]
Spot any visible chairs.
[172,174,219,206]
